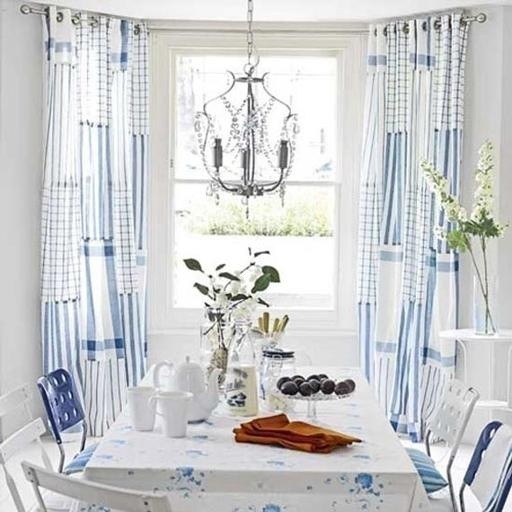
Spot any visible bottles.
[260,349,294,408]
[222,322,257,416]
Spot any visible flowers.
[179,241,280,349]
[417,138,507,335]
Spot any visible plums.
[276,373,355,395]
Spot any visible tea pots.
[152,357,222,424]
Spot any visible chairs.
[1,356,511,511]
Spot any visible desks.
[78,359,431,510]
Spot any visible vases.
[472,271,499,334]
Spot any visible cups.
[124,386,193,440]
